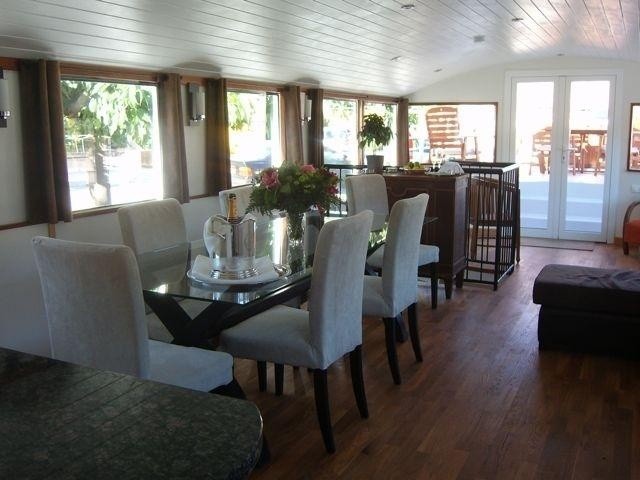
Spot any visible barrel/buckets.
[209,213,256,280]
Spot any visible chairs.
[528,125,608,176]
[425,107,480,163]
[467,175,522,275]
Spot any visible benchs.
[532,264,640,353]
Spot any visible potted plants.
[408,113,419,148]
[357,113,393,175]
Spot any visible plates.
[399,168,427,174]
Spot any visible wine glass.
[430,149,448,169]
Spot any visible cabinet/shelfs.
[345,176,466,299]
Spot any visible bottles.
[226,193,241,222]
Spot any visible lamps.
[0,67,11,128]
[305,93,312,123]
[187,80,206,127]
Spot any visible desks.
[0,345,264,480]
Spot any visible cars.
[231,141,352,182]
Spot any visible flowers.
[244,160,343,240]
